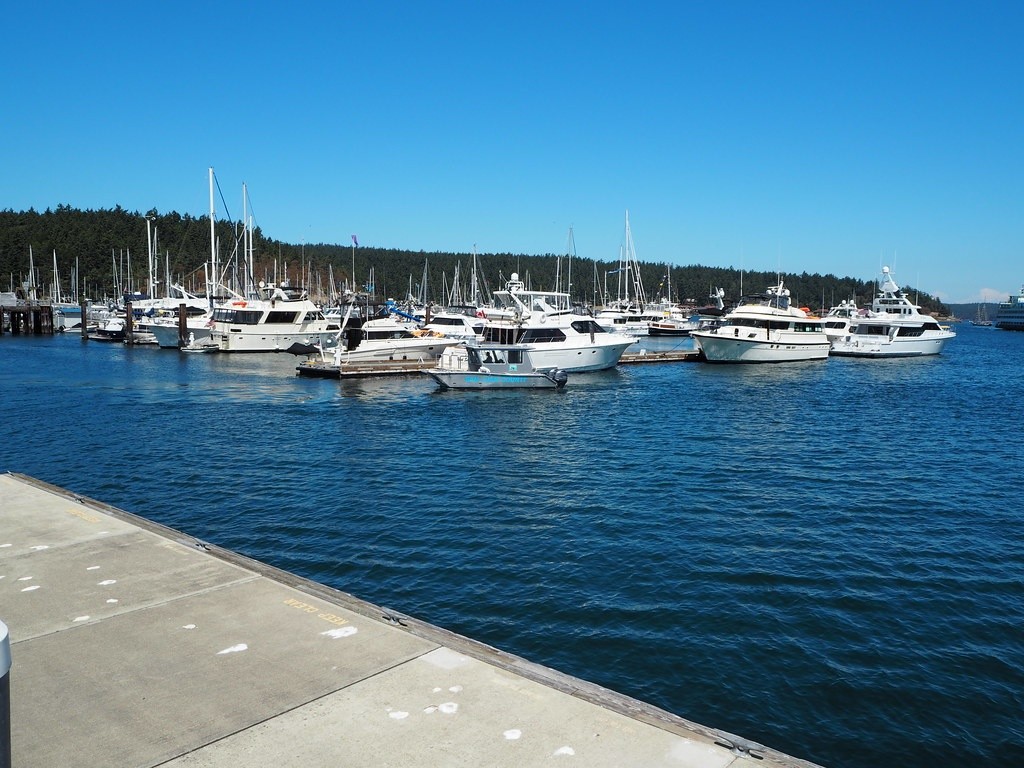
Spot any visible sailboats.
[11,167,729,378]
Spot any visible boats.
[994,288,1024,331]
[417,339,571,388]
[688,277,833,363]
[819,266,957,356]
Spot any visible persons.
[482,352,492,363]
